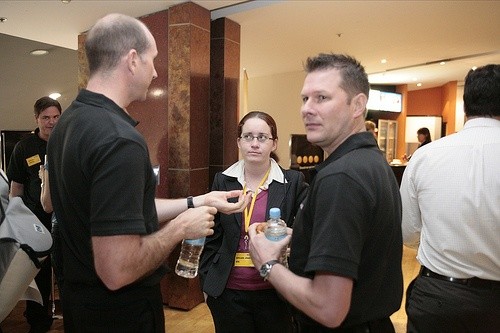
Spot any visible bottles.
[175,237,205,278]
[264,207,288,269]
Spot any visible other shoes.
[27,317,54,333]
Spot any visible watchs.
[259,260,280,282]
[41,182,45,188]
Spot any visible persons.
[7,96,62,333]
[399,64,500,333]
[248,54,404,333]
[197,110,309,333]
[46,12,252,333]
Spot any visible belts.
[422,270,500,289]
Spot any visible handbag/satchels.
[0,196,54,326]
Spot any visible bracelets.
[187,196,195,209]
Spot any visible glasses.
[239,135,274,142]
